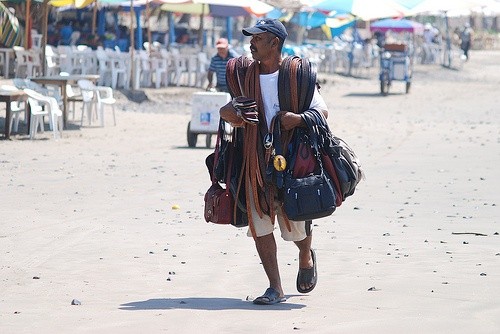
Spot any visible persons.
[455,23,472,60]
[208,38,238,93]
[220,18,328,303]
[47,20,130,51]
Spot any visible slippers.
[253,287,286,304]
[296,248,317,294]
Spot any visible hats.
[242,19,288,41]
[216,38,228,49]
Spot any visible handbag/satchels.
[205,183,232,228]
[282,107,362,221]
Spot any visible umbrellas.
[52,0,414,36]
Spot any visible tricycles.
[371,18,414,95]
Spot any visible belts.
[225,55,317,240]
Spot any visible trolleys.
[186,88,232,148]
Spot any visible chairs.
[0,42,376,139]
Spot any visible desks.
[0,90,32,139]
[32,74,99,130]
